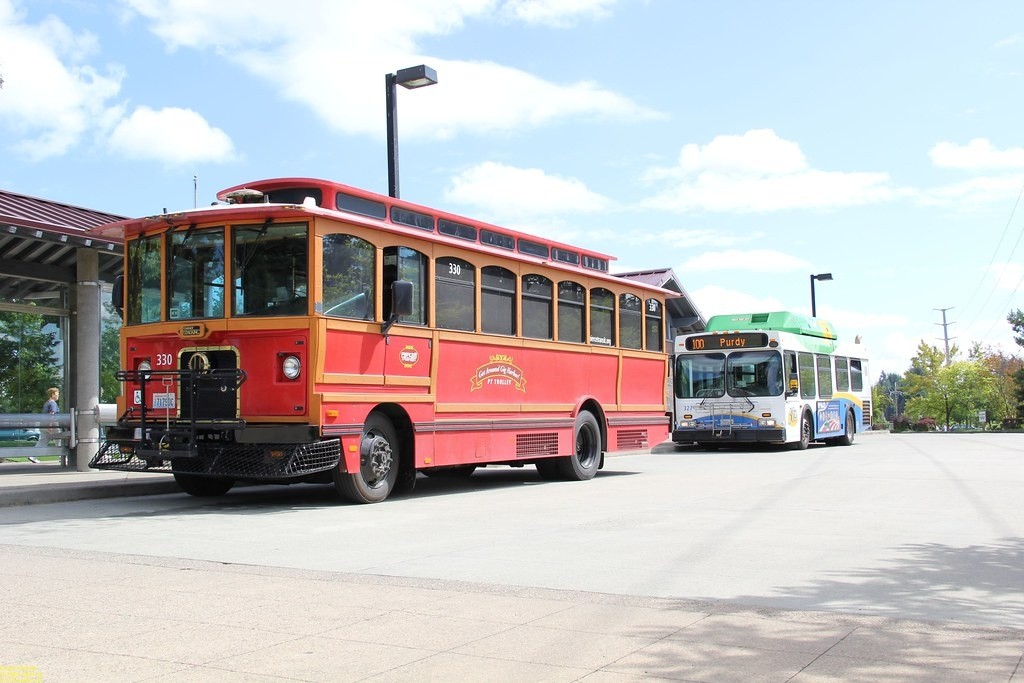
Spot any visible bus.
[667,311,873,452]
[81,176,684,505]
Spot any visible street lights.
[810,272,834,318]
[386,64,439,199]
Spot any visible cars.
[951,425,977,432]
[0,427,40,443]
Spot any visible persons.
[27,387,70,463]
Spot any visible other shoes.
[28,456,41,464]
